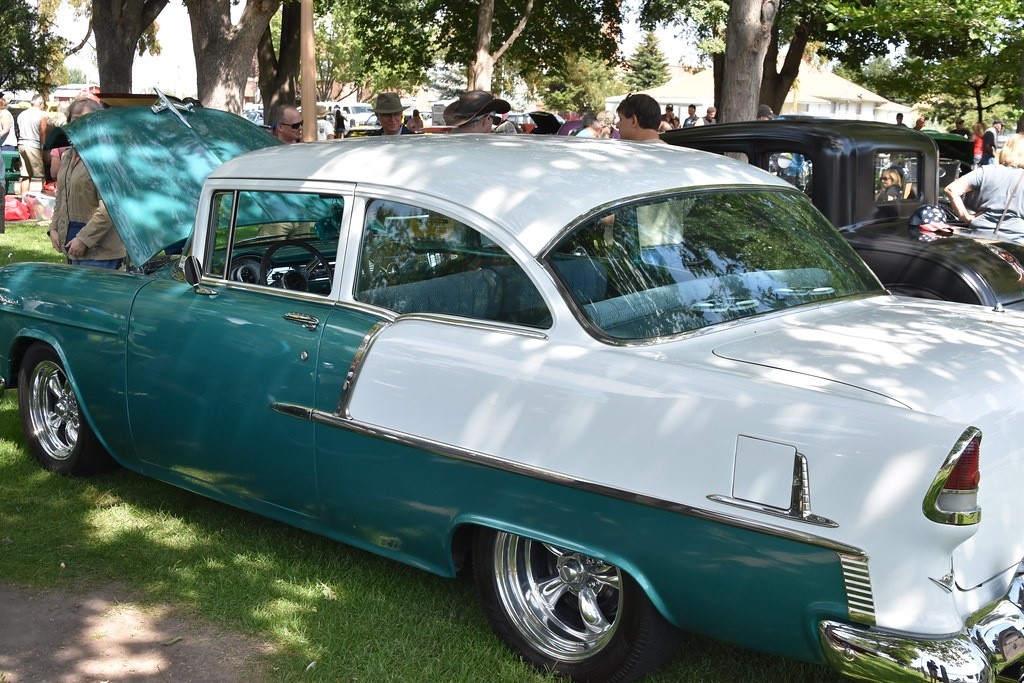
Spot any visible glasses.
[280,120,303,129]
[880,177,892,181]
[484,115,501,125]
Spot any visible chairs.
[360,269,503,322]
[490,256,608,327]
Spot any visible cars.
[0,86,1024,683]
[660,116,1024,311]
[245,100,382,136]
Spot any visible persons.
[575,110,620,139]
[950,113,1024,165]
[694,108,717,127]
[47,99,127,269]
[780,153,805,190]
[661,103,680,130]
[879,153,901,180]
[442,90,511,134]
[366,92,415,136]
[913,119,926,131]
[598,93,697,269]
[683,104,699,128]
[267,98,302,143]
[876,169,902,201]
[896,113,907,127]
[944,134,1024,245]
[757,104,777,120]
[406,105,423,131]
[333,108,348,138]
[316,105,334,141]
[0,95,69,199]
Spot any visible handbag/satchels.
[4,196,29,221]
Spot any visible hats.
[443,90,511,126]
[666,104,674,110]
[912,204,954,233]
[316,106,328,118]
[956,118,964,123]
[993,120,1006,126]
[758,104,777,119]
[368,93,411,113]
